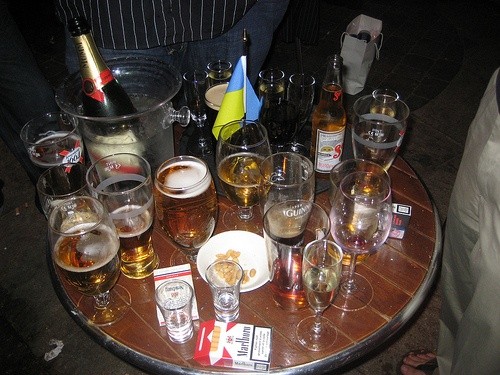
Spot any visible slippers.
[397,350,438,375]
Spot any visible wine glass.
[278,72,316,156]
[47,196,131,324]
[156,155,218,280]
[183,68,216,157]
[295,239,337,352]
[329,171,393,310]
[217,119,274,235]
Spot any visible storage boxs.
[195,319,272,372]
[153,263,199,326]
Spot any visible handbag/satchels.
[340,14,383,95]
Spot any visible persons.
[0,4,60,184]
[396,66,500,375]
[55,0,288,109]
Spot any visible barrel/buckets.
[56,61,181,181]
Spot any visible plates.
[197,229,272,291]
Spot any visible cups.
[154,280,194,345]
[206,260,243,324]
[21,52,410,279]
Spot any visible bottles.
[309,54,349,183]
[69,16,154,190]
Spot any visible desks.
[46,101,443,375]
